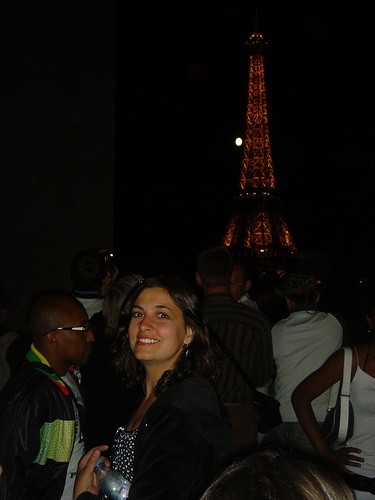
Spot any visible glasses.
[229,278,241,291]
[41,324,91,339]
[133,272,146,285]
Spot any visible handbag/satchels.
[317,401,355,450]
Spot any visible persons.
[291,292,375,500]
[102,274,235,500]
[68,246,354,432]
[74,443,357,500]
[0,288,97,500]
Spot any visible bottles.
[93,459,132,500]
